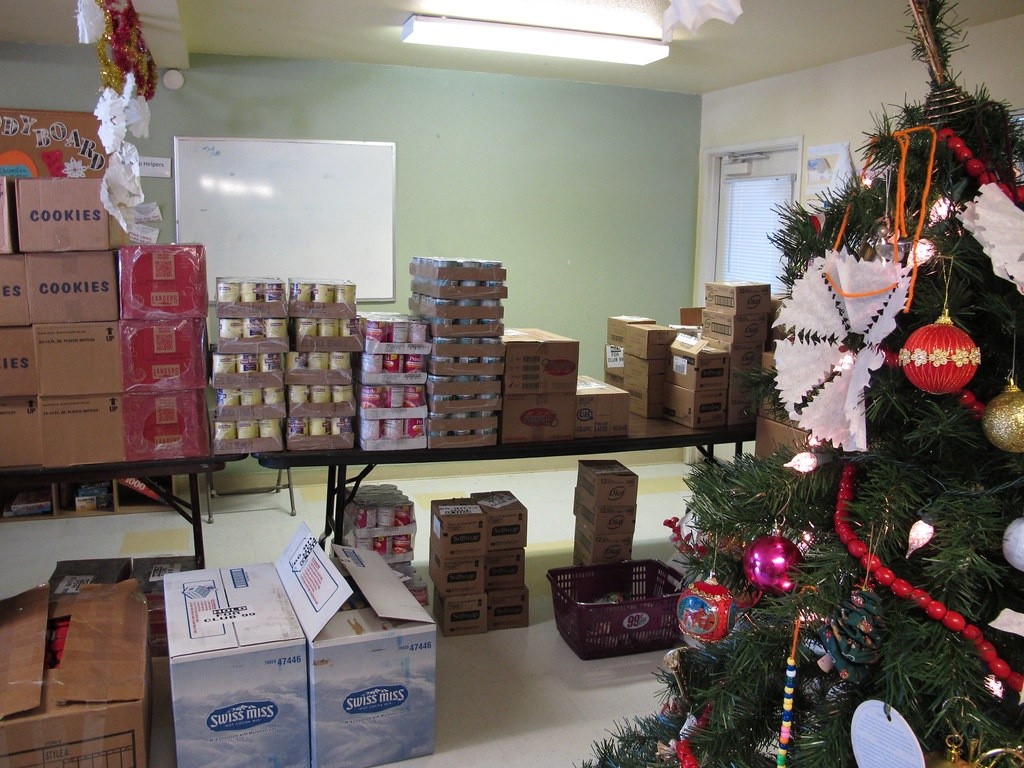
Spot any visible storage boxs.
[3,489,51,518]
[0,175,211,470]
[344,460,688,660]
[59,481,111,511]
[213,263,815,455]
[0,521,436,768]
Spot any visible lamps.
[402,14,669,66]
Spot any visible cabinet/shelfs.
[0,475,177,523]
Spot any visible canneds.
[344,484,415,557]
[359,257,502,440]
[210,275,357,440]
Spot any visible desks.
[250,414,757,558]
[0,434,248,569]
